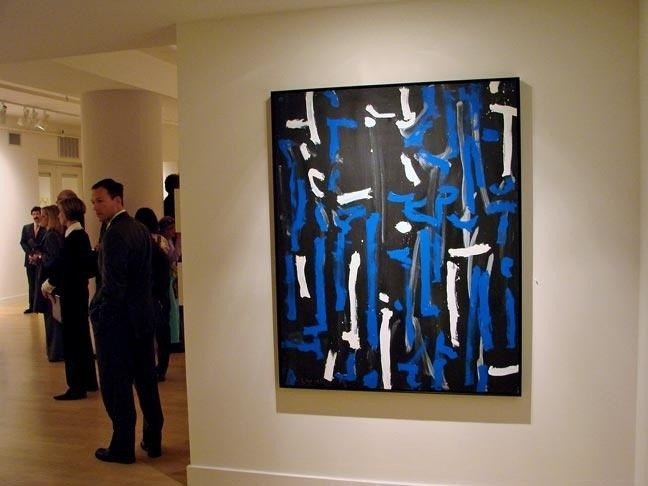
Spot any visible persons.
[85,179,168,465]
[19,173,181,402]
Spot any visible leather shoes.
[141,440,160,457]
[96,448,134,464]
[53,388,85,400]
[23,309,34,313]
